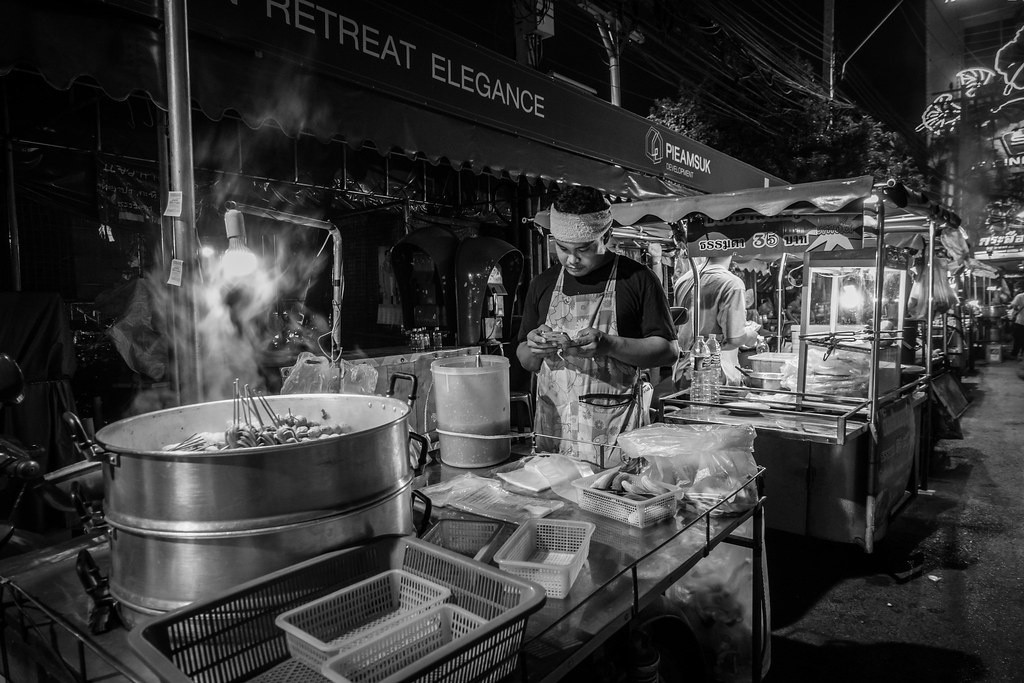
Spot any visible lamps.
[218,200,346,393]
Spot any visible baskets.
[319,602,491,683]
[125,536,548,683]
[415,516,506,565]
[492,516,597,601]
[570,467,683,530]
[272,567,453,678]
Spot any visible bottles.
[689,336,712,411]
[409,326,430,350]
[433,327,443,348]
[707,334,721,402]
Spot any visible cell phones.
[543,331,570,349]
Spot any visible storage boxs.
[746,351,797,375]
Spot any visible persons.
[1006,282,1024,360]
[673,257,758,405]
[758,274,815,325]
[516,186,680,469]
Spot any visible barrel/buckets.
[431,355,512,467]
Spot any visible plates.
[725,402,770,414]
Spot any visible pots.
[62,373,431,630]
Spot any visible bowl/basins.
[748,372,783,389]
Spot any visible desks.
[0,433,772,683]
